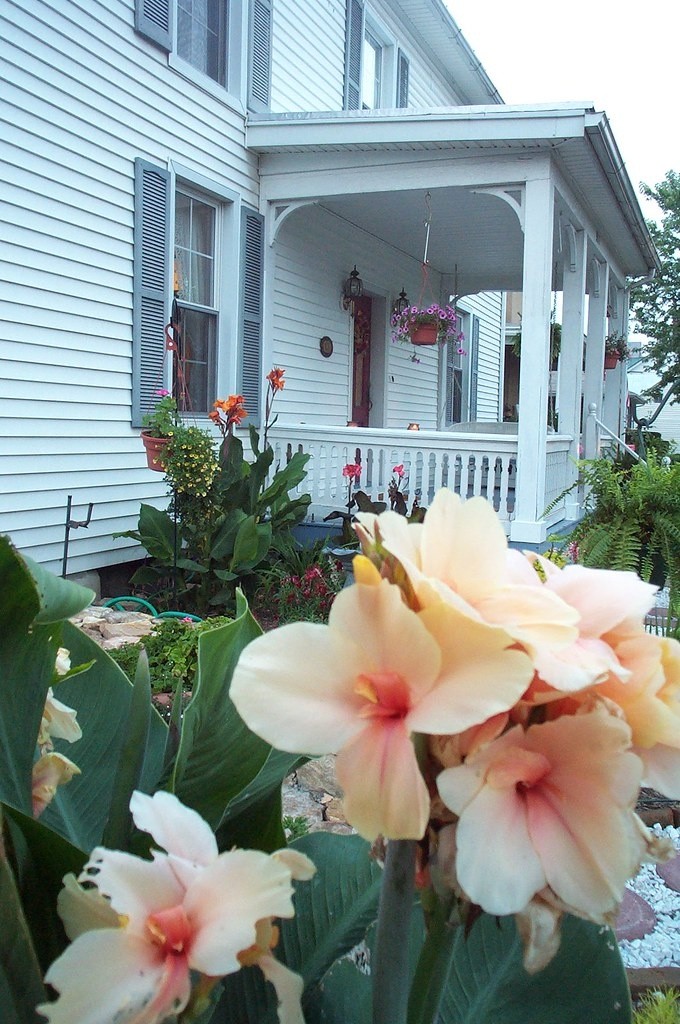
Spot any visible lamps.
[393,286,410,316]
[342,264,367,311]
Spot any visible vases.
[140,431,175,472]
[409,322,438,345]
[604,353,619,369]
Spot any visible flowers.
[604,330,633,359]
[141,388,178,438]
[390,302,467,364]
